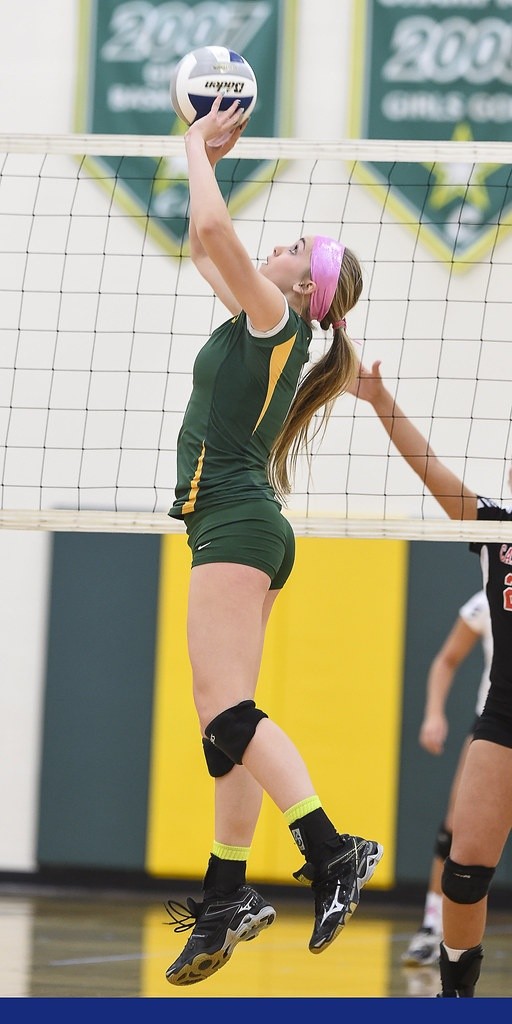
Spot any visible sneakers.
[291,832,384,954]
[162,885,276,986]
[401,927,443,965]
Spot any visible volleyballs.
[170,45,258,132]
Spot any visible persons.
[402,595,485,967]
[166,92,383,987]
[347,360,512,998]
